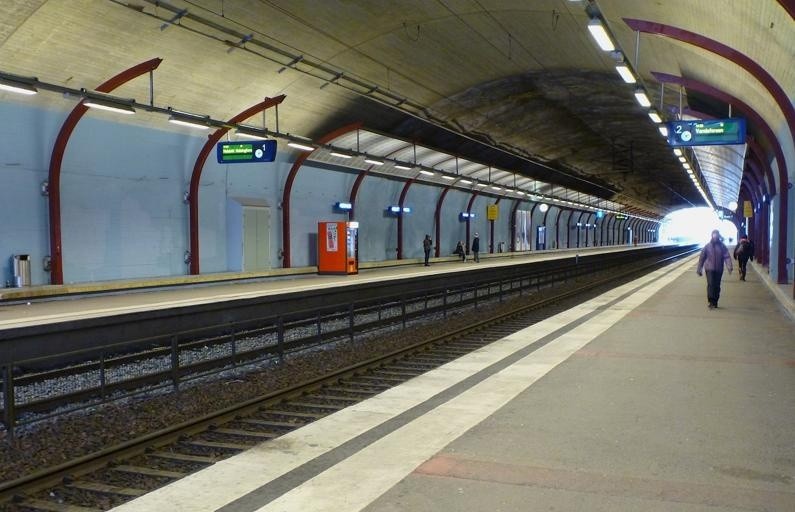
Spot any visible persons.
[696,229,734,310]
[423,234,432,266]
[471,232,480,262]
[457,241,466,261]
[732,234,753,282]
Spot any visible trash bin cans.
[500,241,506,253]
[14,255,31,288]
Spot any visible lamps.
[364,156,385,166]
[0,77,38,95]
[287,138,316,153]
[329,148,353,158]
[79,97,137,115]
[420,167,437,176]
[588,18,723,224]
[393,161,413,172]
[441,174,669,227]
[167,115,210,129]
[235,127,268,142]
